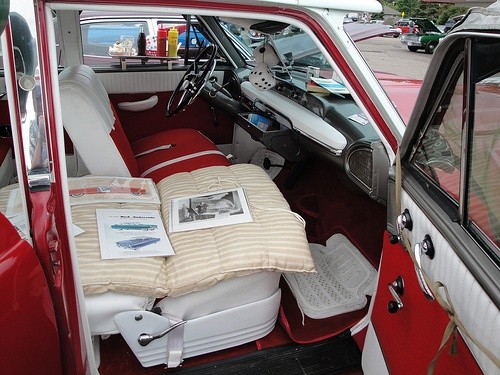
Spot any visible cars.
[0,1,500,375]
[400,18,447,54]
[389,18,420,38]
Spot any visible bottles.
[168,27,178,57]
[305,66,320,86]
[156,28,167,57]
[137,33,146,56]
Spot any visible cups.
[120,36,134,56]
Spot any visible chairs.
[0,164,317,367]
[59,65,232,183]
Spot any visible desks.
[108,52,181,71]
